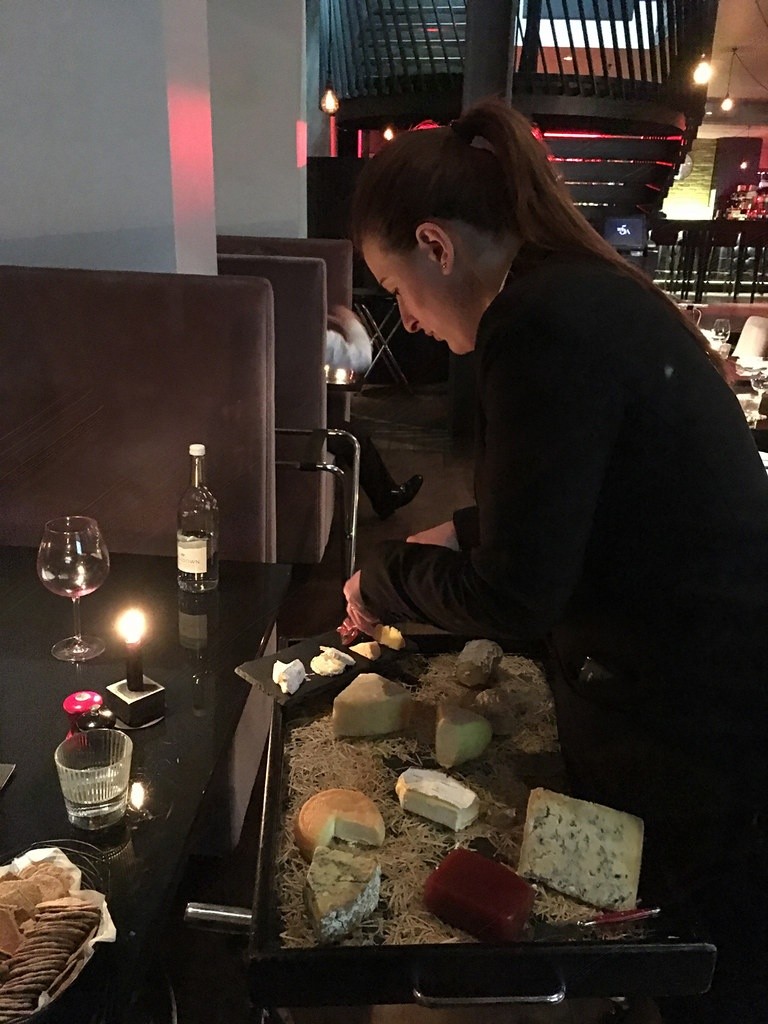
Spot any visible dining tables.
[720,356,768,430]
[0,543,295,1024]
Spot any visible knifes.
[540,904,665,941]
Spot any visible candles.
[114,608,147,691]
[126,782,152,849]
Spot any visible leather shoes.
[372,474,424,518]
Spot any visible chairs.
[344,279,407,396]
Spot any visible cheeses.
[298,639,647,942]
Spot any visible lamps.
[721,48,737,112]
[320,0,339,114]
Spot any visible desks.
[650,218,768,303]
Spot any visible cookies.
[0,862,101,1024]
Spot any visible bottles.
[177,593,217,717]
[176,444,219,593]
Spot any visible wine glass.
[750,370,768,415]
[36,516,110,662]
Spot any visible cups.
[713,318,730,342]
[55,729,132,828]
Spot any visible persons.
[325,306,424,517]
[337,97,768,1024]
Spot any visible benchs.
[0,237,353,564]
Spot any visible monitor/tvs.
[600,214,647,249]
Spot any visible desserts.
[272,623,406,695]
